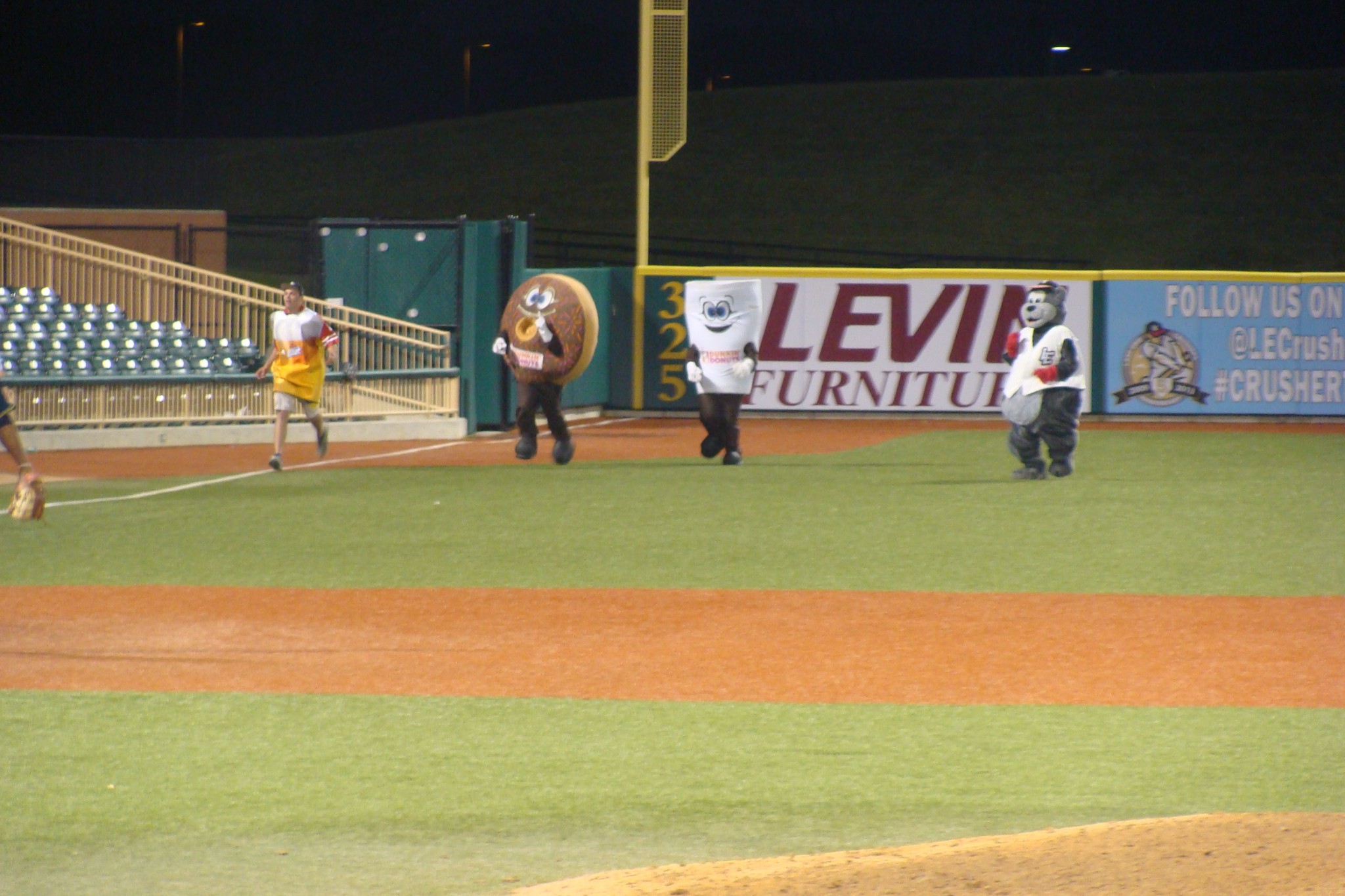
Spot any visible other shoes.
[269,455,282,470]
[316,426,327,455]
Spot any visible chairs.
[0,285,270,405]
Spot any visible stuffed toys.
[491,275,598,465]
[684,279,763,466]
[1000,280,1086,481]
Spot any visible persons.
[0,387,46,523]
[256,281,338,471]
